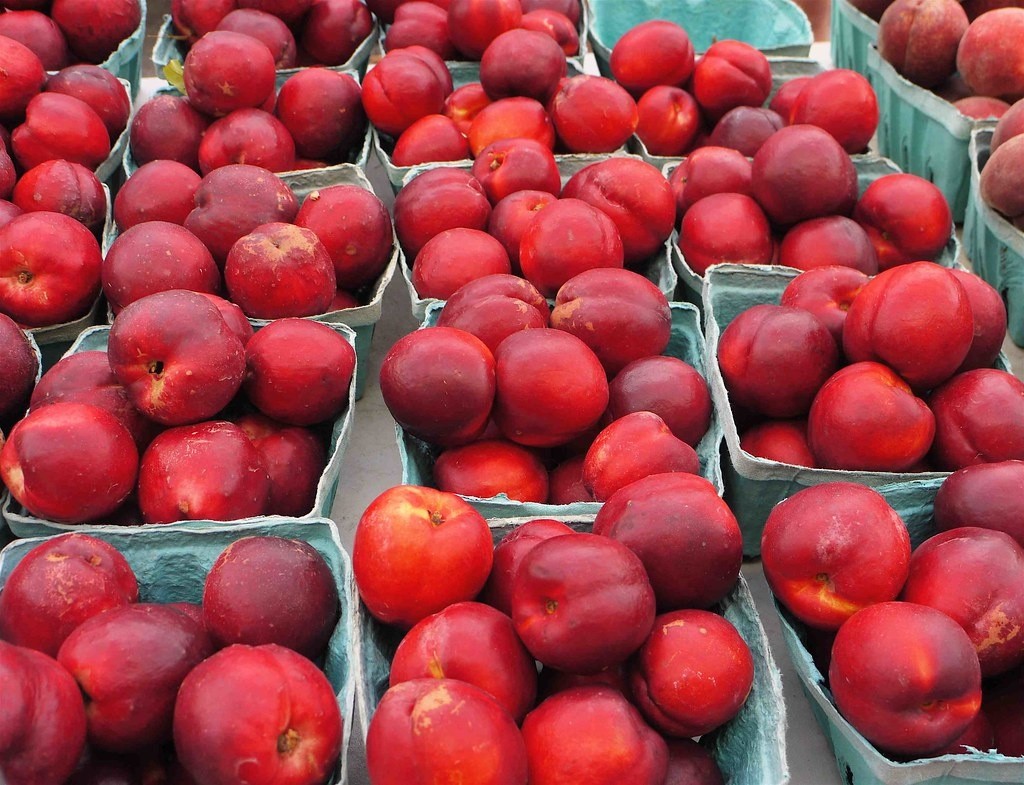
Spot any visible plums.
[0,0,1024,785]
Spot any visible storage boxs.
[0,0,1024,785]
